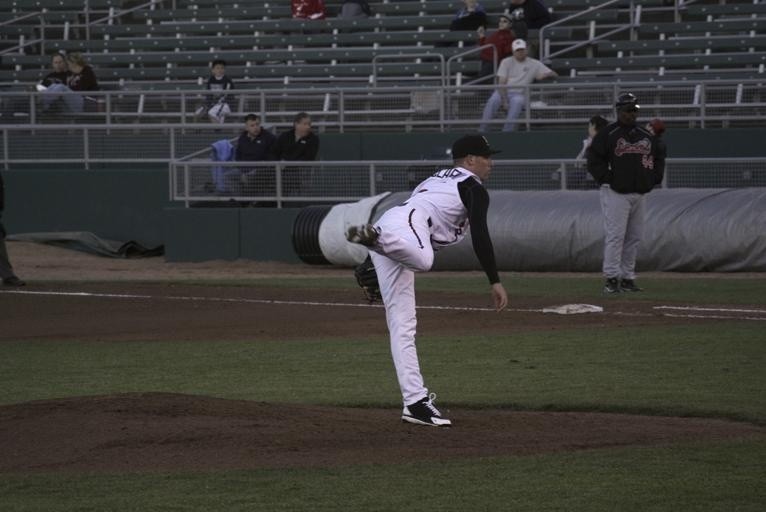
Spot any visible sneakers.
[344,225,379,244]
[402,398,451,428]
[1,276,25,287]
[621,279,642,292]
[604,278,619,292]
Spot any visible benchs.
[0,1,766,138]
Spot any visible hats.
[511,39,526,53]
[453,133,502,159]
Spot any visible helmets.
[616,94,640,112]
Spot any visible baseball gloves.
[356,252,383,302]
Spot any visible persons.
[29,54,72,129]
[345,134,509,425]
[291,1,325,34]
[275,112,320,208]
[40,53,99,146]
[451,0,557,131]
[224,114,275,207]
[195,59,234,134]
[574,94,665,295]
